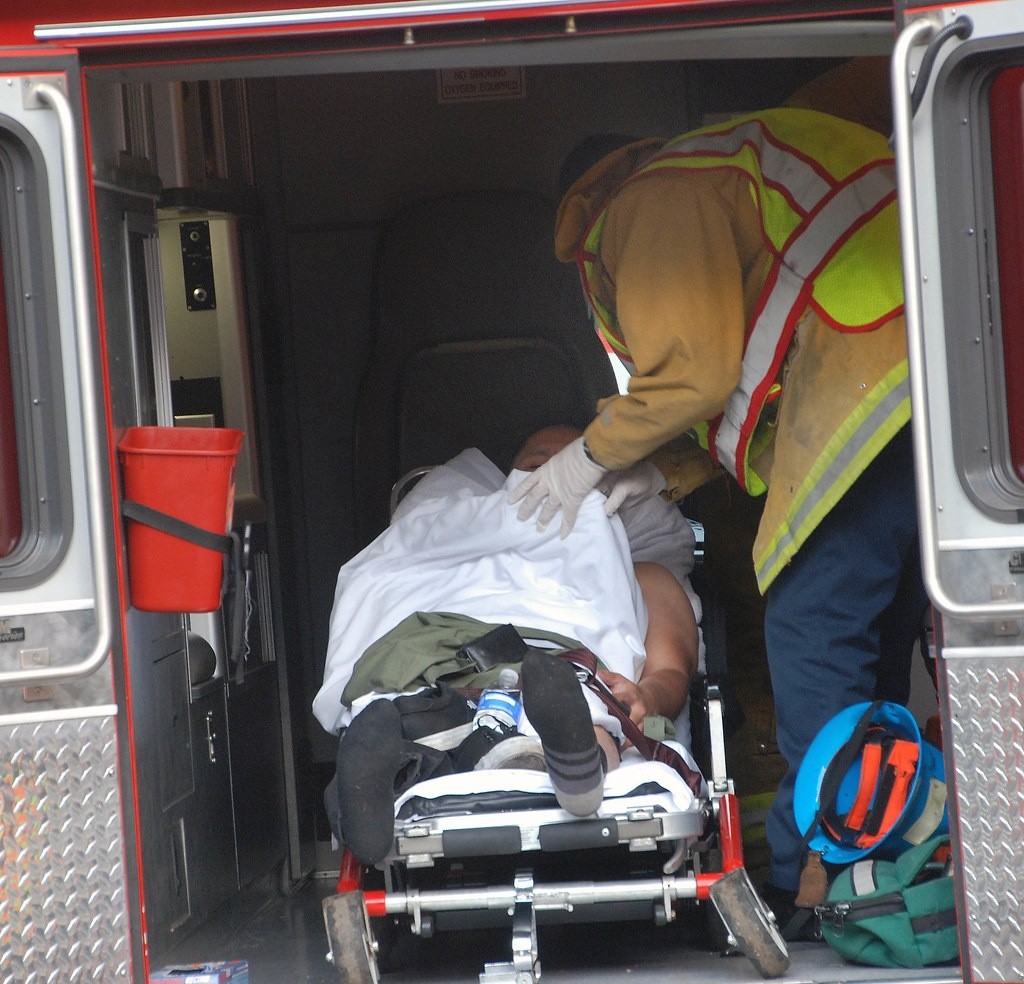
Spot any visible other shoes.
[762,883,816,941]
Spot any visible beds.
[316,460,791,983]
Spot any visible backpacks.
[815,835,961,969]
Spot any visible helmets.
[792,700,949,864]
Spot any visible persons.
[508,110,922,940]
[338,423,697,868]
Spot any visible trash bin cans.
[118,427,245,618]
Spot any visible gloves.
[594,460,666,517]
[507,436,610,540]
